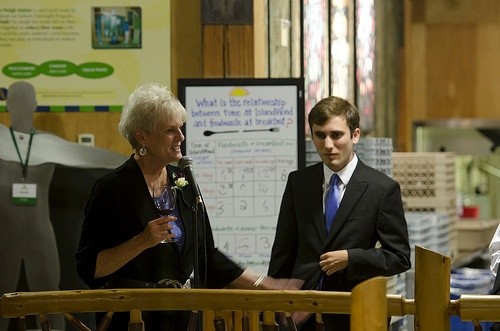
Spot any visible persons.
[488,221,500,294]
[75,82,306,331]
[0,81,132,331]
[267,96,412,331]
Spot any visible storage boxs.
[458,218,499,251]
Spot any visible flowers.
[172,172,189,190]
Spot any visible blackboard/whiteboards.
[175,77,306,275]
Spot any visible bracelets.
[251,276,265,290]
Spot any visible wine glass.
[153,185,179,244]
